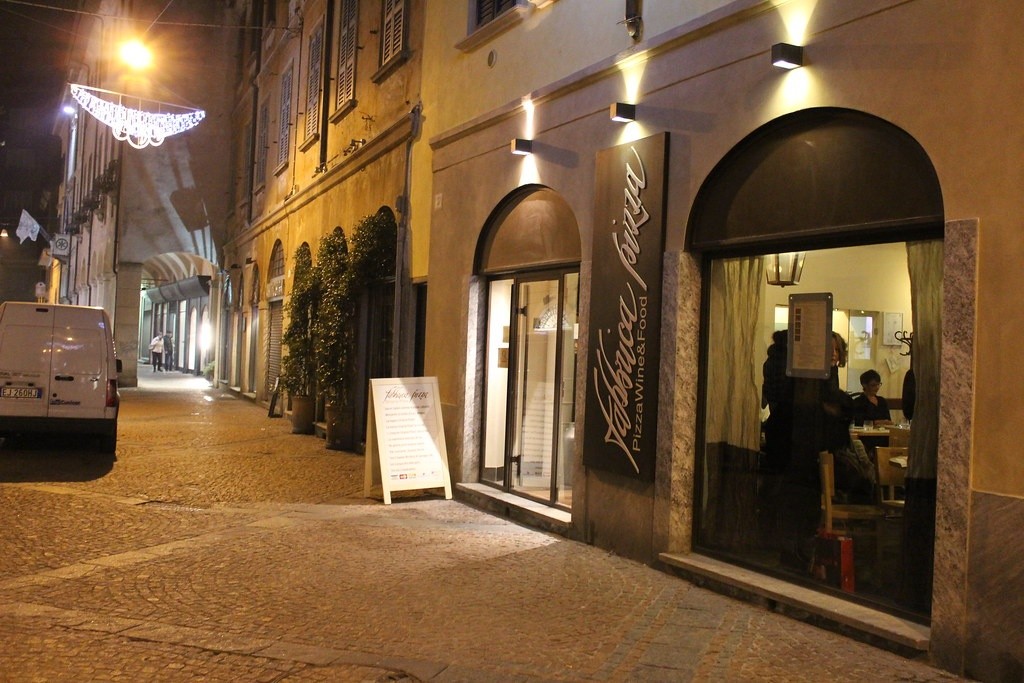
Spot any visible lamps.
[808,449,884,586]
[350,138,367,147]
[314,162,326,175]
[510,138,532,156]
[609,102,635,123]
[764,250,807,289]
[770,42,802,70]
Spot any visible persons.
[151,332,164,372]
[761,328,847,576]
[163,331,174,372]
[853,369,892,447]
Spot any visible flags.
[16,209,40,244]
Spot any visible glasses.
[862,383,883,388]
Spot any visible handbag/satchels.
[148,344,155,350]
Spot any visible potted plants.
[313,227,357,450]
[278,246,325,435]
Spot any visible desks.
[845,426,910,439]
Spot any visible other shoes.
[158,368,162,371]
[153,370,156,372]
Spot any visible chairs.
[874,444,909,559]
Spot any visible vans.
[0,301,123,452]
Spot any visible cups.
[863,421,874,432]
[897,418,910,429]
